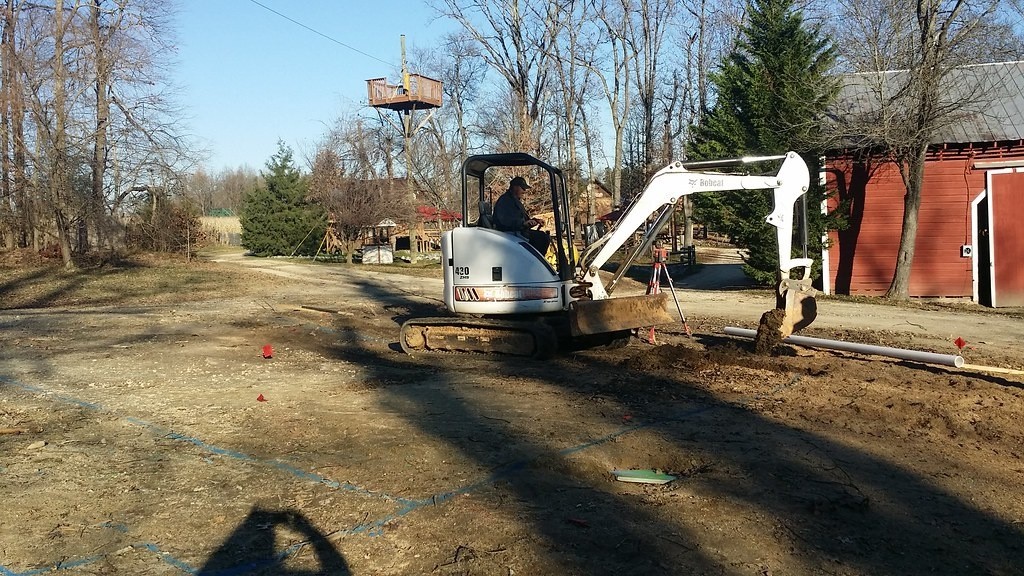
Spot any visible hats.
[510,177,532,189]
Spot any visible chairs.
[478,201,495,229]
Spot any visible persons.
[492,177,550,257]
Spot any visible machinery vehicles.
[398,149,825,364]
[109,185,164,216]
